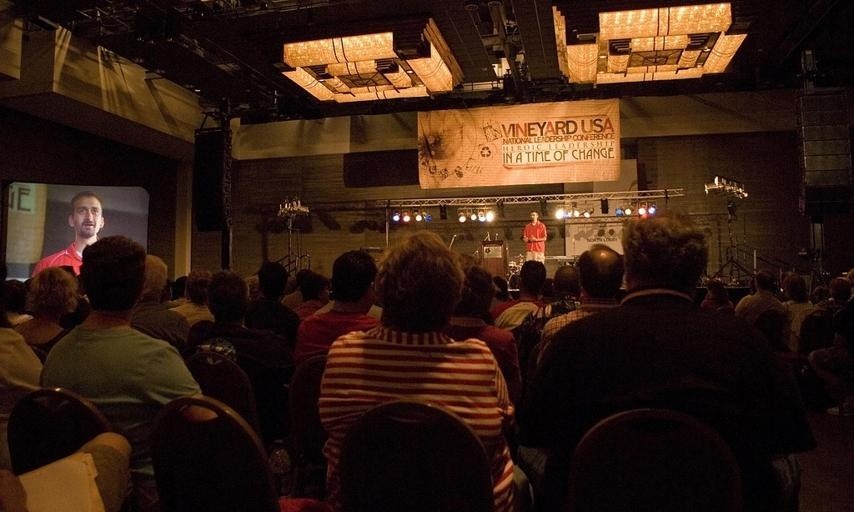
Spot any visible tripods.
[711,205,763,288]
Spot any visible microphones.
[495,231,500,239]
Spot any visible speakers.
[191,129,234,234]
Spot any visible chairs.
[0,277,853,512]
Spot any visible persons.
[0,211,854,512]
[32,193,105,278]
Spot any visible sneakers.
[827,401,854,417]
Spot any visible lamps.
[556,201,595,219]
[455,205,496,223]
[391,207,433,222]
[276,200,310,221]
[704,175,749,201]
[615,199,657,218]
